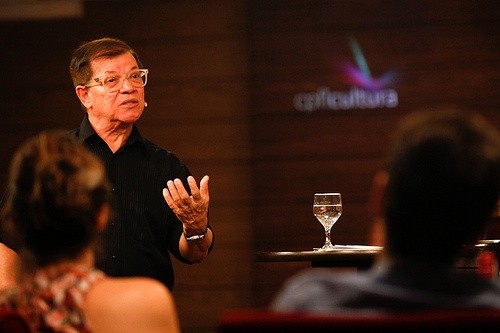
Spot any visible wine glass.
[312,193,343,251]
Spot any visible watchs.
[183,229,210,243]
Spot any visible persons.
[265,103,500,318]
[1,128,182,333]
[66,38,216,302]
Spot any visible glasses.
[83,69,149,92]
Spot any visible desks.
[257,243,500,274]
